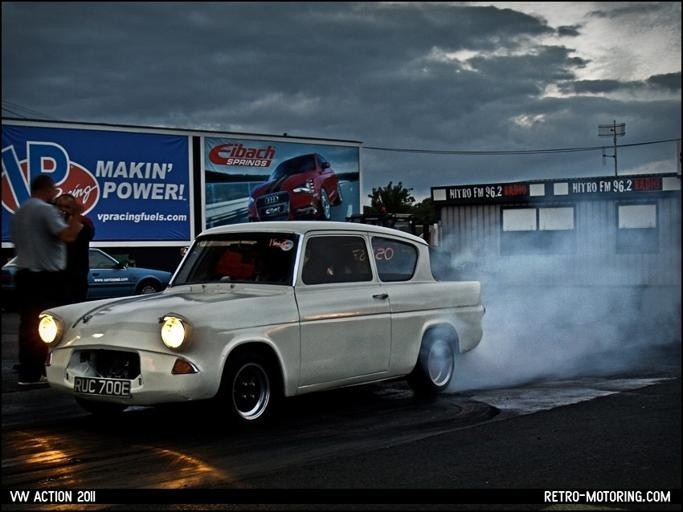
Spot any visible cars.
[2,248,173,313]
[37,220,485,439]
[248,153,344,222]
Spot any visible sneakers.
[17,371,48,385]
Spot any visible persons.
[9,173,84,385]
[51,192,95,305]
